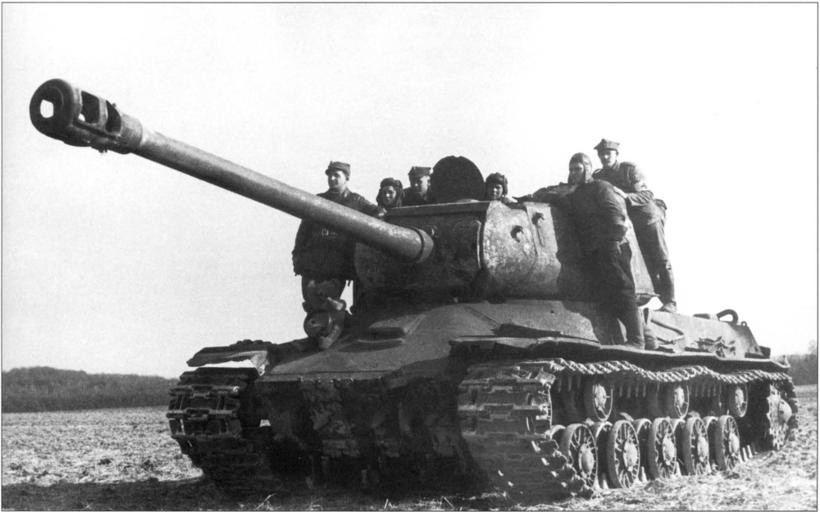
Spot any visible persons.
[484,171,516,204]
[352,177,406,310]
[591,138,677,312]
[517,153,658,350]
[292,162,378,350]
[402,165,436,204]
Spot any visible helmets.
[485,172,507,195]
[376,178,405,206]
[568,153,593,182]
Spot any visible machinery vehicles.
[27,77,800,497]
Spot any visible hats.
[325,161,350,175]
[408,166,431,178]
[594,138,620,151]
